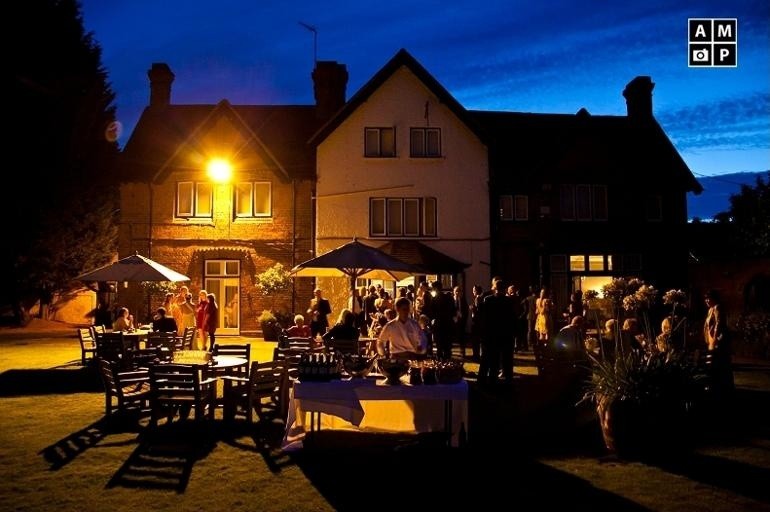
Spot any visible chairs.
[70,296,735,483]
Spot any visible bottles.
[407,361,464,384]
[297,353,342,382]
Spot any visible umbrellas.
[72,249,191,332]
[287,236,422,329]
[375,239,465,298]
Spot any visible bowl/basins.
[378,357,412,380]
[342,355,375,379]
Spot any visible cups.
[172,350,212,361]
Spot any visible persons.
[84,286,220,351]
[288,275,736,388]
